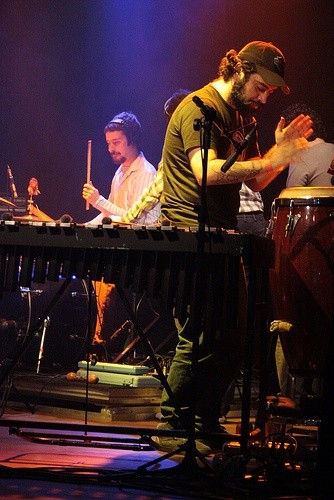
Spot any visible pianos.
[0,217,243,456]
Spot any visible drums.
[272,185,334,377]
[0,256,98,368]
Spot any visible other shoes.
[159,436,211,455]
[196,427,241,453]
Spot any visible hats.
[237,41,290,95]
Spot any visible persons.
[69,90,334,369]
[149,42,313,458]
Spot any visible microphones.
[7,166,18,199]
[221,125,257,173]
